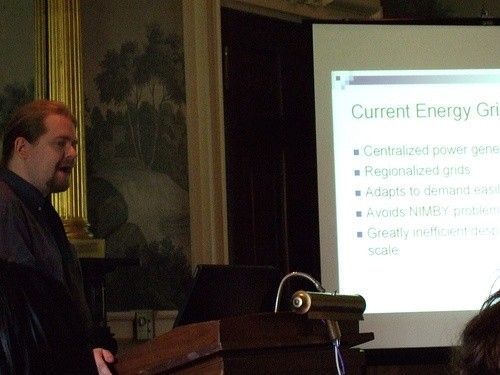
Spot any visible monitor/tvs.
[173,264,276,329]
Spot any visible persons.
[0,100,115,375]
